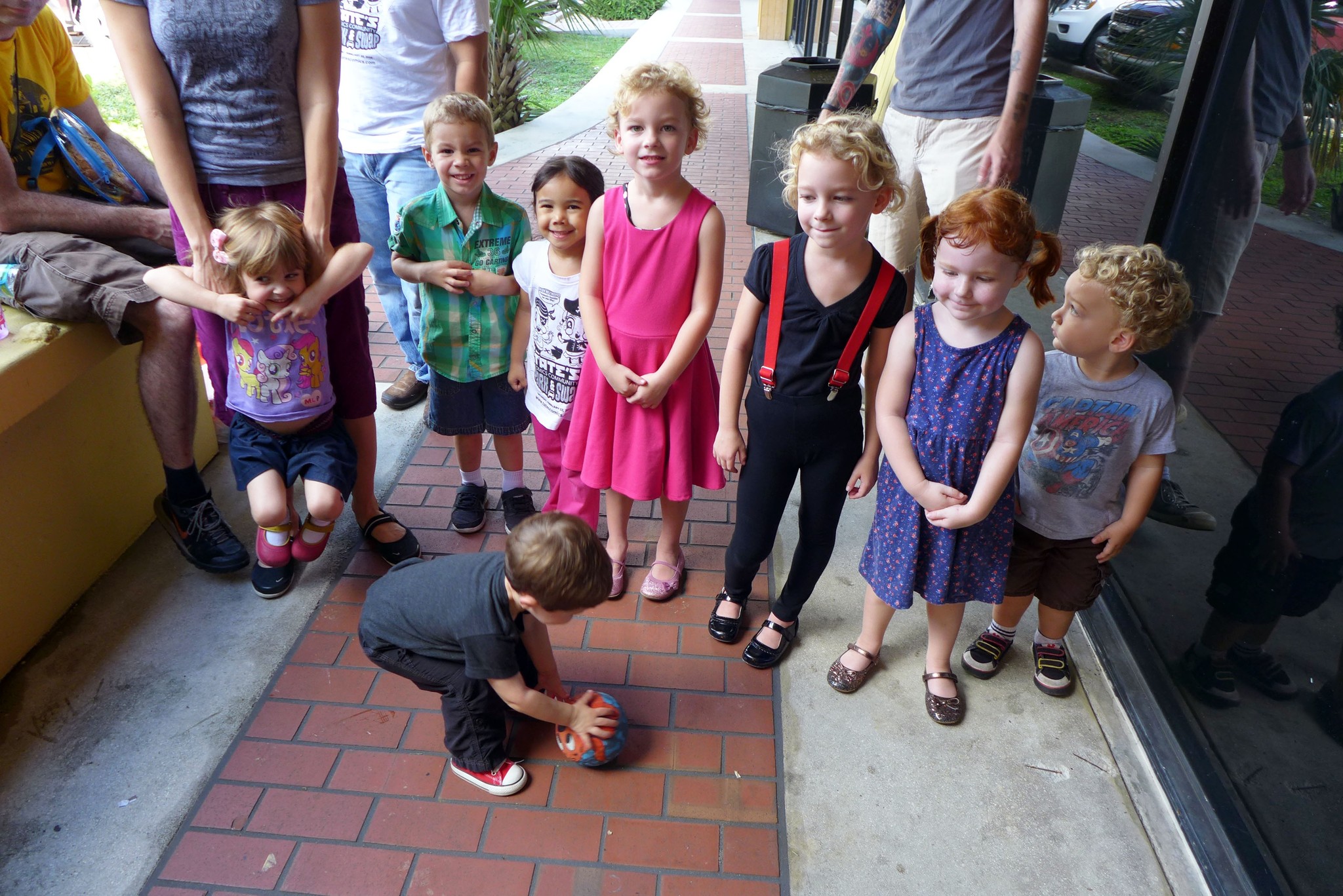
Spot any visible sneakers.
[1181,643,1242,707]
[501,486,537,536]
[154,487,251,574]
[961,632,1014,680]
[451,755,527,796]
[1230,643,1291,686]
[450,478,488,533]
[1030,642,1072,695]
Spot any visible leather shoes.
[382,368,428,408]
[742,611,799,668]
[708,586,747,643]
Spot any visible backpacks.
[21,106,150,206]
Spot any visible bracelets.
[821,101,840,114]
[1279,137,1312,150]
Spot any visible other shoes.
[827,642,881,693]
[922,667,962,724]
[1138,478,1219,532]
[255,525,292,568]
[358,505,422,566]
[640,546,685,601]
[291,512,336,563]
[605,548,625,599]
[249,514,303,599]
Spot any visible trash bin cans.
[1015,73,1093,236]
[746,57,877,238]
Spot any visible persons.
[357,512,616,795]
[707,111,909,671]
[384,91,537,540]
[1174,371,1343,710]
[959,239,1190,696]
[1121,0,1325,535]
[561,64,729,600]
[806,0,1042,318]
[823,190,1066,725]
[98,0,425,570]
[143,198,376,566]
[1,0,274,572]
[505,154,613,545]
[328,0,501,409]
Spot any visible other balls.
[555,692,628,767]
